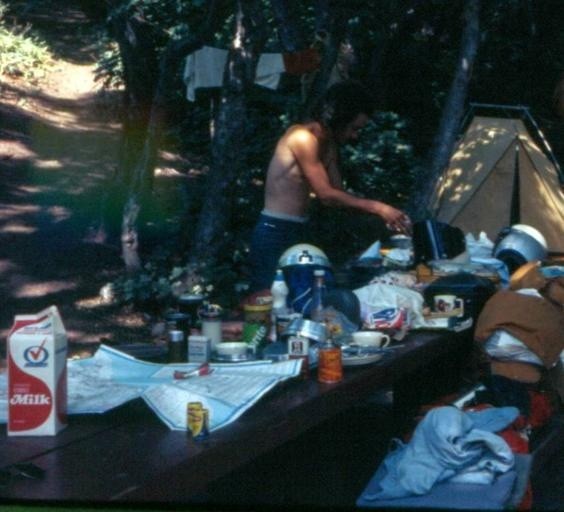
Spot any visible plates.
[340,344,385,367]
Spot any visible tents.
[432,111,564,260]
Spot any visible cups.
[351,330,391,349]
[216,341,257,356]
[433,293,465,319]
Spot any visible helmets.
[279,243,334,272]
[493,223,547,272]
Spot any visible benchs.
[528,426,564,477]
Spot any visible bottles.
[166,328,186,364]
[317,338,345,385]
[310,269,328,324]
[267,274,290,344]
[199,310,223,354]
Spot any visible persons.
[252,79,415,295]
[183,33,227,142]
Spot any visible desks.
[1,330,468,502]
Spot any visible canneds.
[242,303,272,349]
[164,311,191,340]
[186,401,209,442]
[178,294,204,321]
